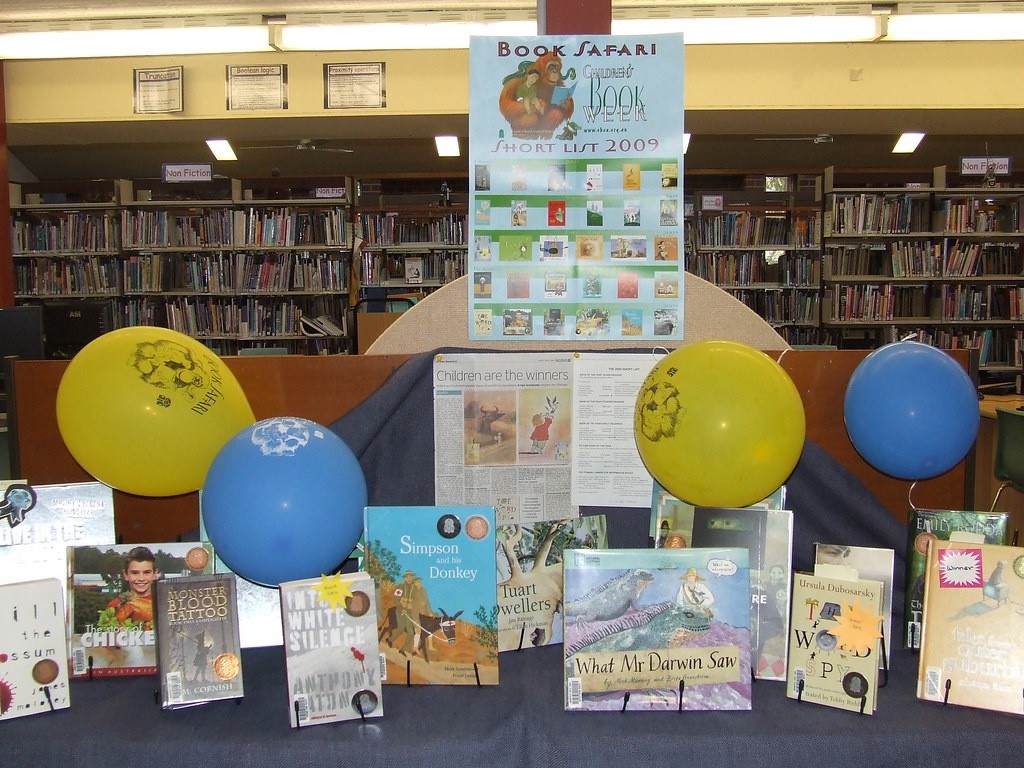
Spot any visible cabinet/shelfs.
[9,190,1024,395]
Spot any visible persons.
[510,310,527,327]
[479,275,485,292]
[477,174,485,187]
[662,178,675,187]
[513,213,519,226]
[658,282,673,294]
[106,547,158,626]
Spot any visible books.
[903,507,1010,650]
[0,579,71,721]
[66,542,219,679]
[0,482,117,625]
[12,207,470,354]
[197,486,895,728]
[154,573,245,710]
[684,192,1024,395]
[915,538,1024,714]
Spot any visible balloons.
[633,340,806,509]
[202,417,368,588]
[844,341,981,480]
[55,326,257,497]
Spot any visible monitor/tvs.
[44,298,115,349]
